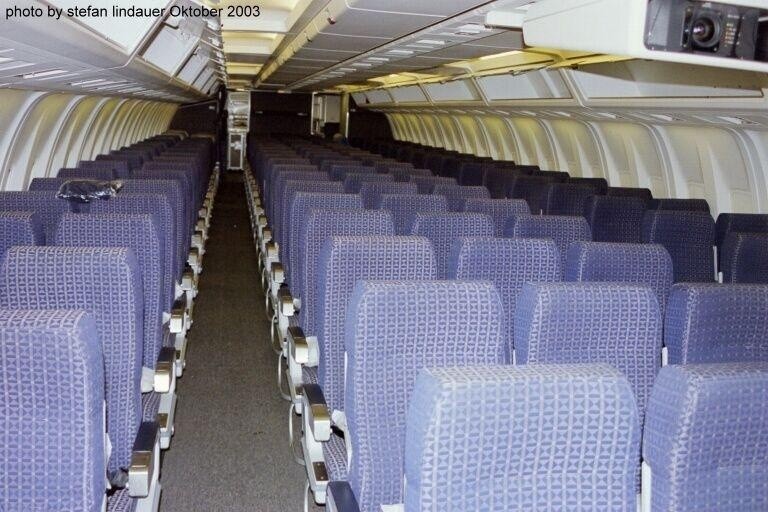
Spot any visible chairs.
[515,282,663,482]
[456,153,475,162]
[652,197,712,214]
[455,197,533,237]
[483,169,528,199]
[389,168,432,182]
[376,195,451,240]
[369,136,410,162]
[317,140,384,163]
[511,176,559,215]
[0,209,48,250]
[663,285,768,367]
[469,156,493,166]
[715,233,768,284]
[321,160,364,172]
[568,242,674,381]
[243,136,264,256]
[409,143,446,170]
[505,214,595,273]
[513,165,541,177]
[106,179,187,378]
[0,247,157,512]
[380,365,638,512]
[53,212,177,448]
[374,161,415,172]
[262,179,346,292]
[545,184,595,217]
[78,160,130,178]
[485,160,514,169]
[265,208,411,411]
[258,170,328,274]
[279,236,438,502]
[29,178,70,190]
[714,213,768,272]
[565,177,609,195]
[439,151,460,177]
[459,163,493,187]
[57,165,116,181]
[0,189,72,245]
[451,239,564,365]
[329,164,377,180]
[268,194,390,363]
[131,169,195,332]
[96,154,142,170]
[530,170,569,184]
[140,128,220,177]
[408,174,458,195]
[327,282,507,512]
[0,309,108,512]
[431,184,491,213]
[109,143,141,156]
[359,181,418,209]
[261,132,318,179]
[343,172,395,194]
[607,186,652,207]
[408,214,496,281]
[190,166,219,275]
[641,363,768,512]
[641,210,720,288]
[582,195,656,251]
